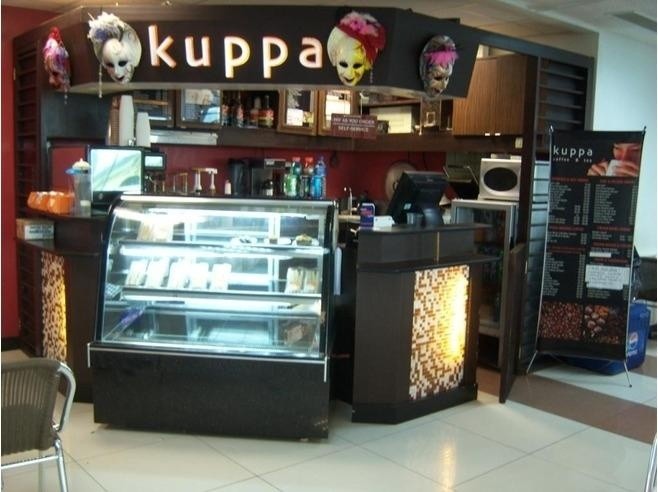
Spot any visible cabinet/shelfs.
[112,89,278,130]
[452,54,528,137]
[88,193,339,441]
[277,87,358,137]
[361,94,452,140]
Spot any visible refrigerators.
[451,198,548,377]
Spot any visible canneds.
[360,202,375,228]
[284,174,323,198]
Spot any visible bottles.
[288,155,327,200]
[220,90,275,130]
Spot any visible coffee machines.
[229,157,287,200]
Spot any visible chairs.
[1,359,76,492]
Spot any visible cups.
[66,158,92,217]
[606,160,625,177]
[110,94,151,148]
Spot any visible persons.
[586,143,641,177]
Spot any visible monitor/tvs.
[388,170,449,223]
[84,143,146,205]
